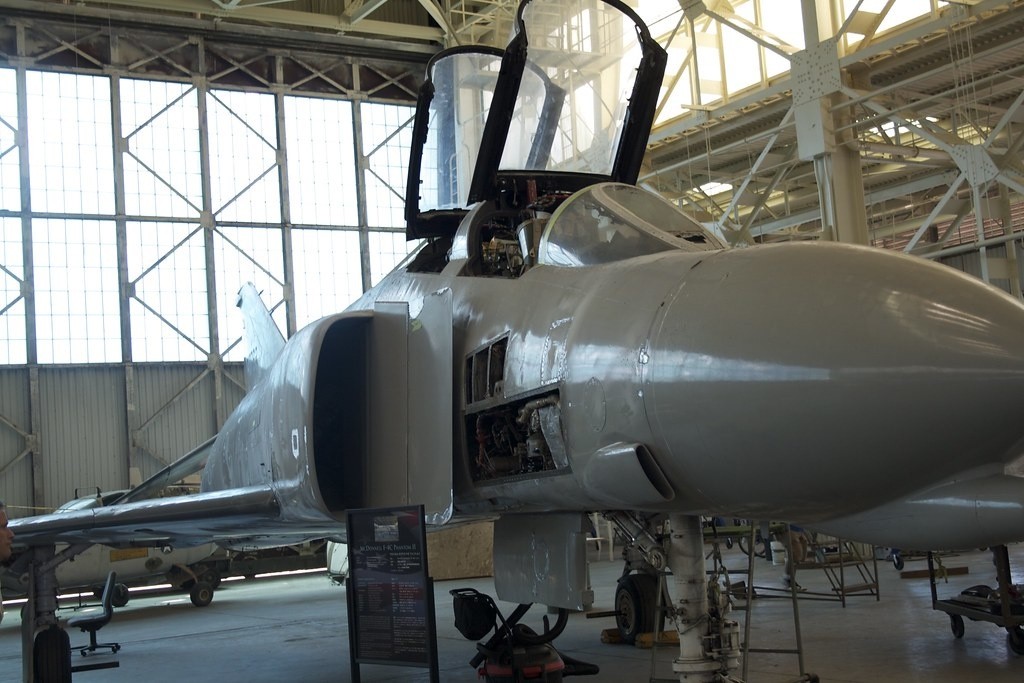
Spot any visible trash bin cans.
[32,622,73,683]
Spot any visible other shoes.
[787,583,807,591]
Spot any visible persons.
[772,521,808,592]
[0,501,15,630]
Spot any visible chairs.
[66,569,120,658]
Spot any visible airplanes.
[0,1,1024,646]
